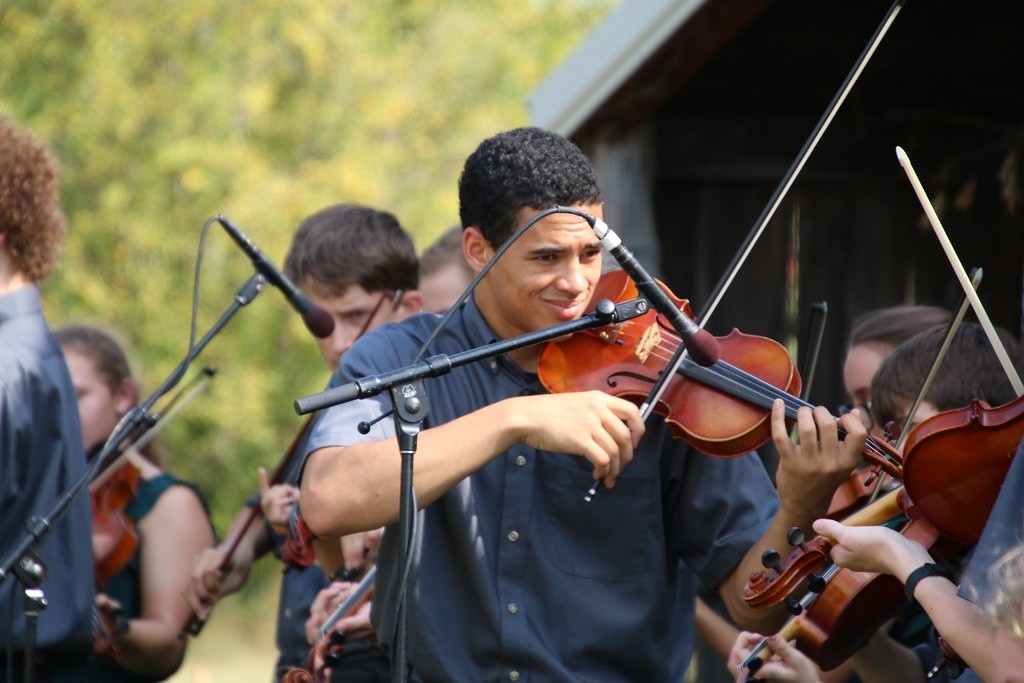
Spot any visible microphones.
[588,216,720,367]
[220,216,335,339]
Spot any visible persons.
[51,324,219,683]
[300,128,871,683]
[697,316,1024,682]
[843,302,964,470]
[2,114,98,682]
[183,204,425,683]
[418,224,475,314]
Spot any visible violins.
[744,389,1024,683]
[84,444,141,654]
[539,265,904,484]
[263,425,319,575]
[280,562,377,683]
